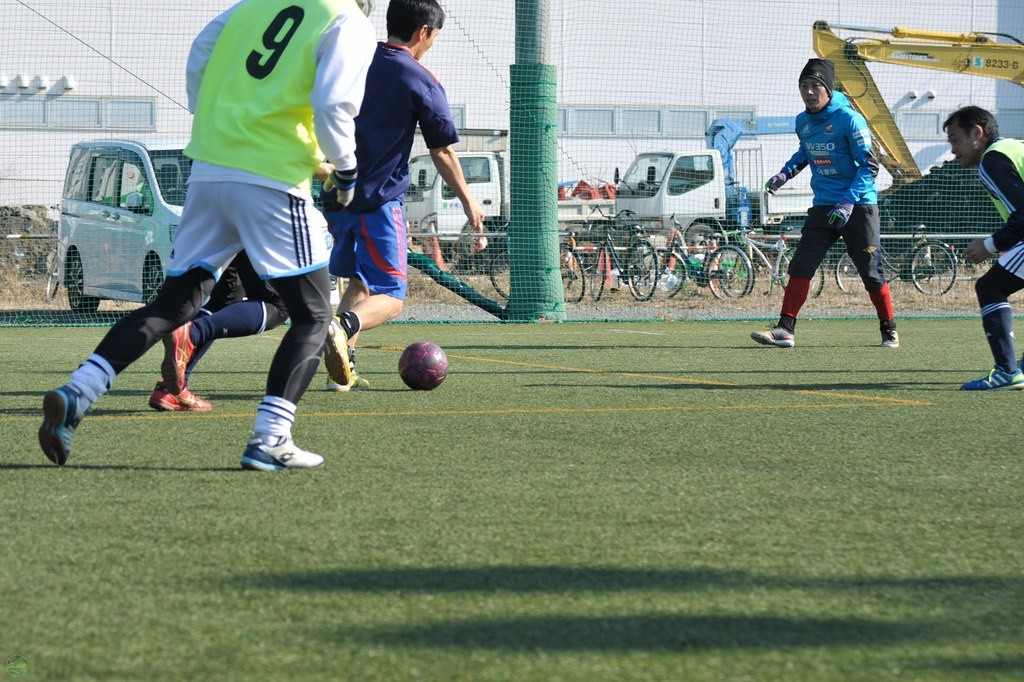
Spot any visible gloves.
[323,171,358,207]
[765,173,786,195]
[826,198,854,228]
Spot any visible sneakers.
[961,365,1024,390]
[326,370,368,392]
[322,316,351,385]
[241,436,324,472]
[38,386,84,465]
[149,381,212,411]
[161,321,195,395]
[751,324,795,347]
[880,323,899,348]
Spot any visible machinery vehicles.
[811,18,1024,202]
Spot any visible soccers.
[398,340,449,391]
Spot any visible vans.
[57,135,211,316]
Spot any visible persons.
[326,0,484,391]
[38,0,378,470]
[751,58,900,348]
[942,106,1024,390]
[149,250,290,412]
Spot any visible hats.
[799,58,834,96]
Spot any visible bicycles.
[835,208,959,295]
[631,211,824,302]
[490,222,586,302]
[589,203,659,302]
[45,250,59,303]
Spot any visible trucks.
[612,105,955,249]
[403,151,618,274]
